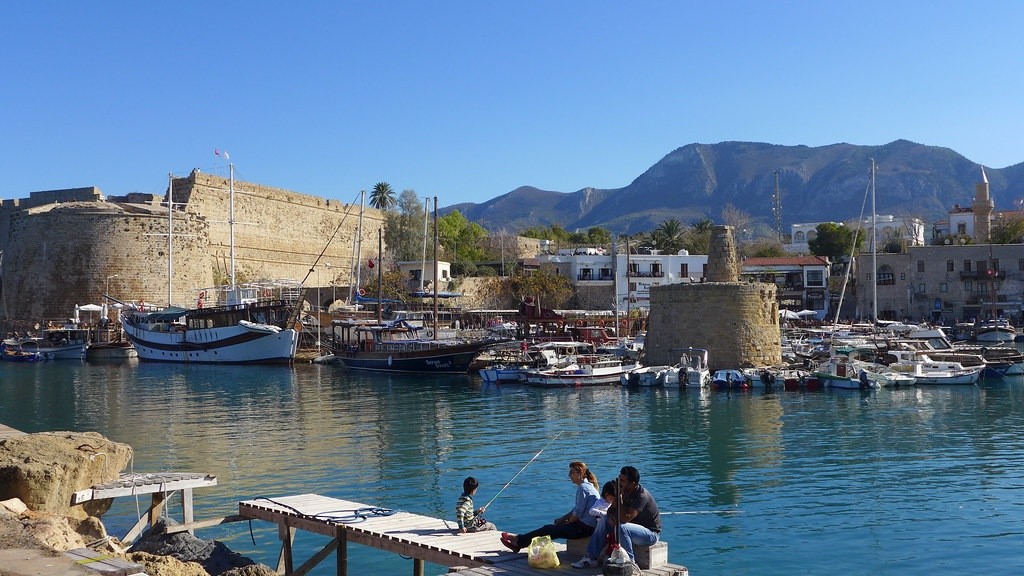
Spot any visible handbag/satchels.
[527,535,560,569]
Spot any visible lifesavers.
[200,292,204,298]
[359,289,365,295]
[140,299,144,306]
[427,331,432,337]
[566,348,574,354]
[981,348,988,354]
[622,320,627,326]
[577,320,582,326]
[599,320,604,326]
[197,303,202,308]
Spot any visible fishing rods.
[465,428,566,528]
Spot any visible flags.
[215,149,229,160]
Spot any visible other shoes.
[502,533,509,540]
[500,537,520,553]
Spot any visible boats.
[0,159,1024,392]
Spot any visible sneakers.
[570,556,598,568]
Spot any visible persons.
[521,338,528,361]
[451,316,502,331]
[570,466,661,567]
[99,318,113,329]
[500,461,601,552]
[455,476,497,533]
[192,167,200,172]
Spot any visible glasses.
[569,470,579,474]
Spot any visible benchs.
[65,546,144,576]
[491,354,518,357]
[567,535,668,569]
[591,360,623,368]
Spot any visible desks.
[495,349,519,361]
